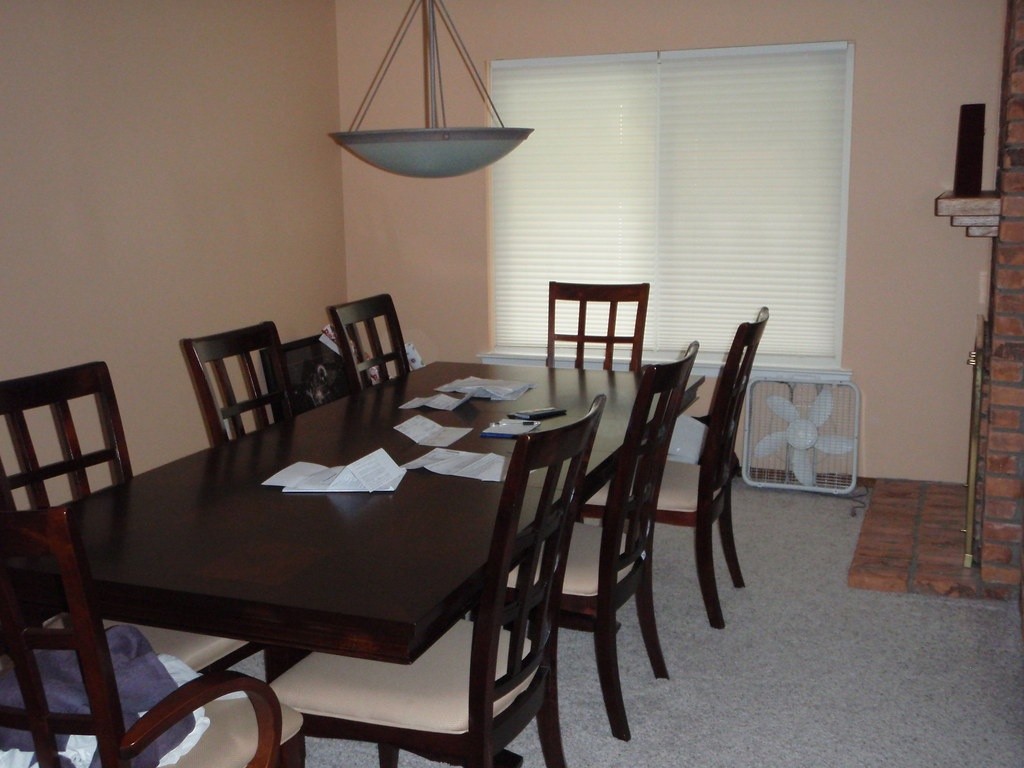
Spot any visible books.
[507,408,567,418]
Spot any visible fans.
[740,378,861,494]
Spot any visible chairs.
[178,319,287,446]
[502,338,702,742]
[0,360,265,673]
[326,293,410,393]
[270,393,607,768]
[0,507,282,768]
[581,306,769,630]
[545,282,650,371]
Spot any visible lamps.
[328,0,535,177]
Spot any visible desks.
[0,361,707,768]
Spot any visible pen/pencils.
[492,421,538,427]
